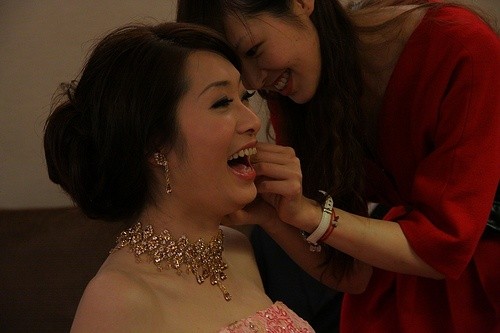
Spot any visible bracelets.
[309,205,337,252]
[302,195,333,244]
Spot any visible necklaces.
[110,223,231,301]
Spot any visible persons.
[43,22,317,332]
[175,0,500,332]
